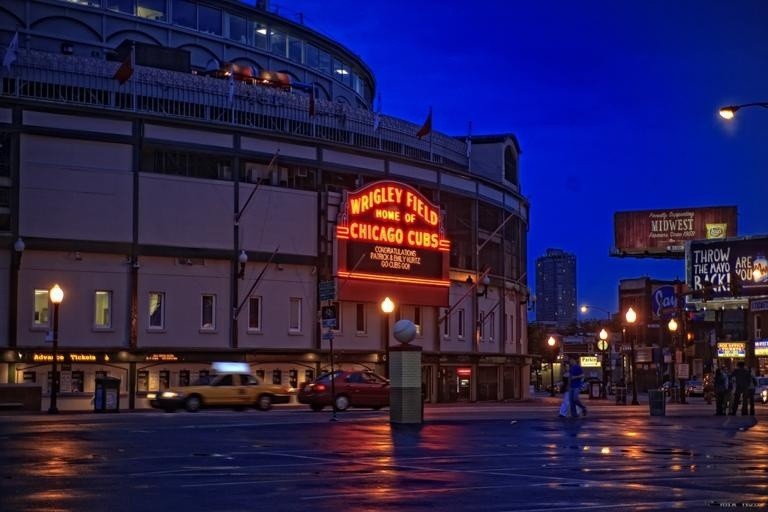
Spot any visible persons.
[715,361,755,416]
[568,359,588,418]
[558,367,584,416]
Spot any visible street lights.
[382,295,395,379]
[626,306,641,405]
[546,335,558,398]
[579,304,614,393]
[719,102,768,121]
[44,284,66,412]
[597,326,611,400]
[666,281,694,405]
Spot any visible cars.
[545,376,633,396]
[299,369,391,412]
[657,376,768,404]
[147,360,292,413]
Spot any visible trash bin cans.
[589,382,602,399]
[94,377,119,411]
[648,391,664,416]
